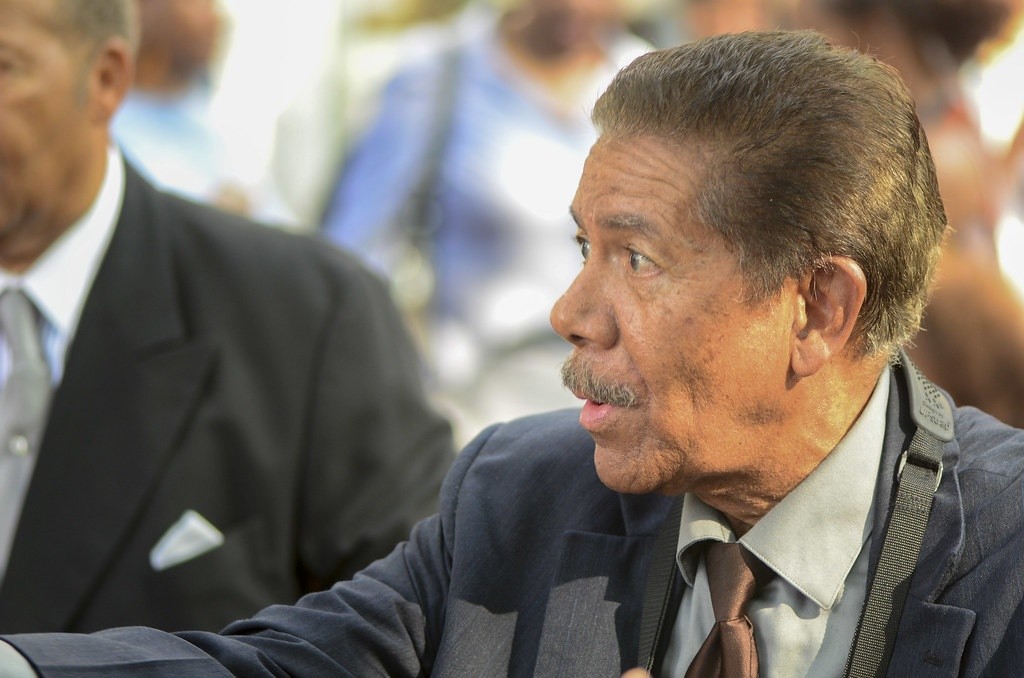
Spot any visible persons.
[2,0,457,635]
[308,1,657,451]
[1,29,1024,678]
[110,2,323,233]
[793,2,1022,427]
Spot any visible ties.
[684,540,776,678]
[0,289,53,588]
